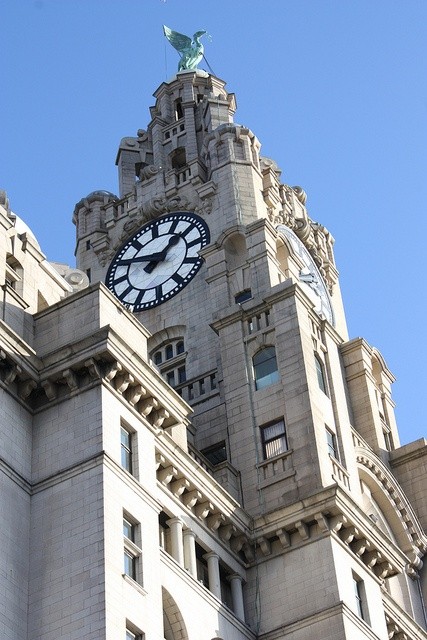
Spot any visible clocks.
[275,222,335,327]
[101,212,211,313]
